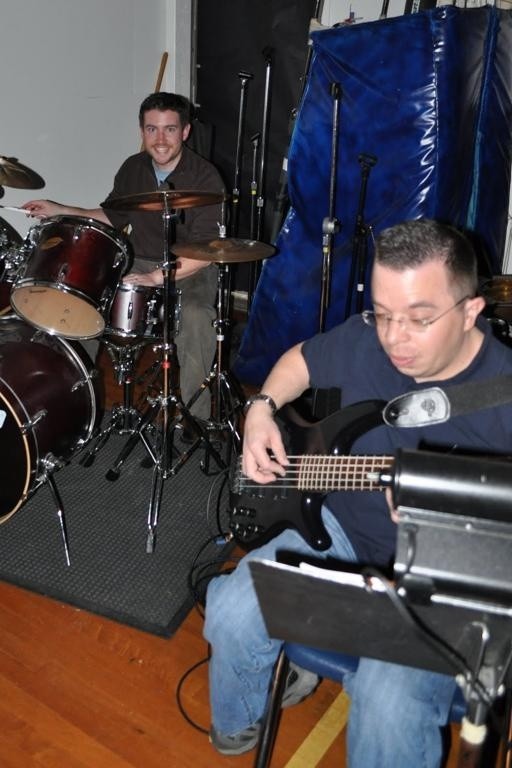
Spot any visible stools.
[254,640,490,768]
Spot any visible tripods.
[80,326,185,471]
[104,207,248,555]
[141,262,247,479]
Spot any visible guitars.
[228,398,400,552]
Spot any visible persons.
[203,215,511,766]
[17,91,227,449]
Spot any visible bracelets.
[242,391,278,420]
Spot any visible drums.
[110,282,180,342]
[1,312,105,524]
[12,214,135,341]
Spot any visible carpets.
[0,410,238,640]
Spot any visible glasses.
[359,294,472,336]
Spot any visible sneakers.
[203,654,326,760]
[177,416,227,448]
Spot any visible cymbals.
[169,235,277,262]
[0,150,46,189]
[99,189,240,211]
